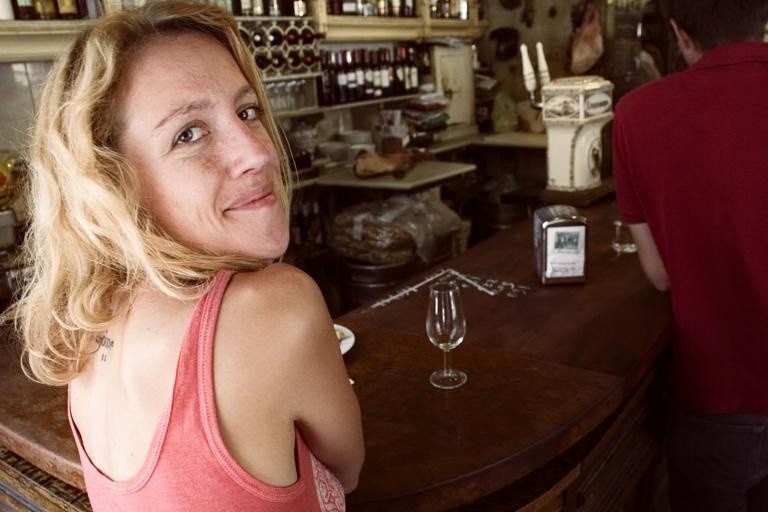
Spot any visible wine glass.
[425,282,468,390]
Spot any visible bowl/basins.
[350,144,376,159]
[318,141,343,153]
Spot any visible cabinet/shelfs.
[1,0,487,191]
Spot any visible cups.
[609,203,639,254]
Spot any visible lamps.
[520,43,616,206]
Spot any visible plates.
[339,130,373,145]
[333,322,356,357]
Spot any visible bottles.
[239,29,326,69]
[316,46,419,107]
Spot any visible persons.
[605,0,768,512]
[6,0,368,512]
